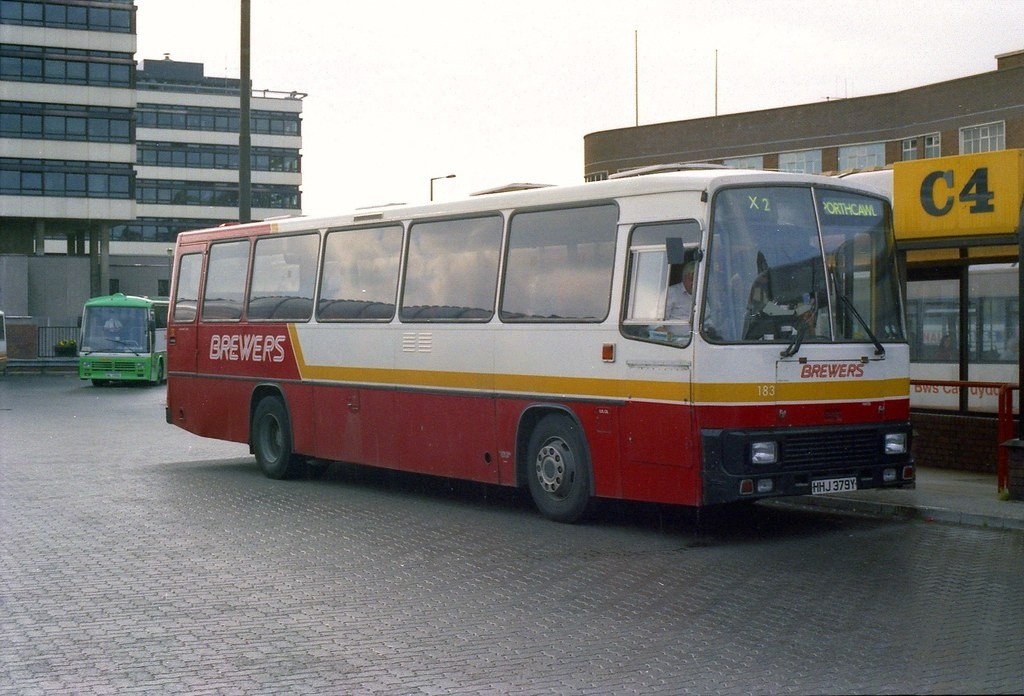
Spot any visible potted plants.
[54,339,79,357]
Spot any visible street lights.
[429,174,457,201]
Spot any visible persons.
[765,230,833,338]
[935,335,952,357]
[104,314,122,332]
[649,262,715,340]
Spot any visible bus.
[167,164,919,528]
[77,294,169,388]
